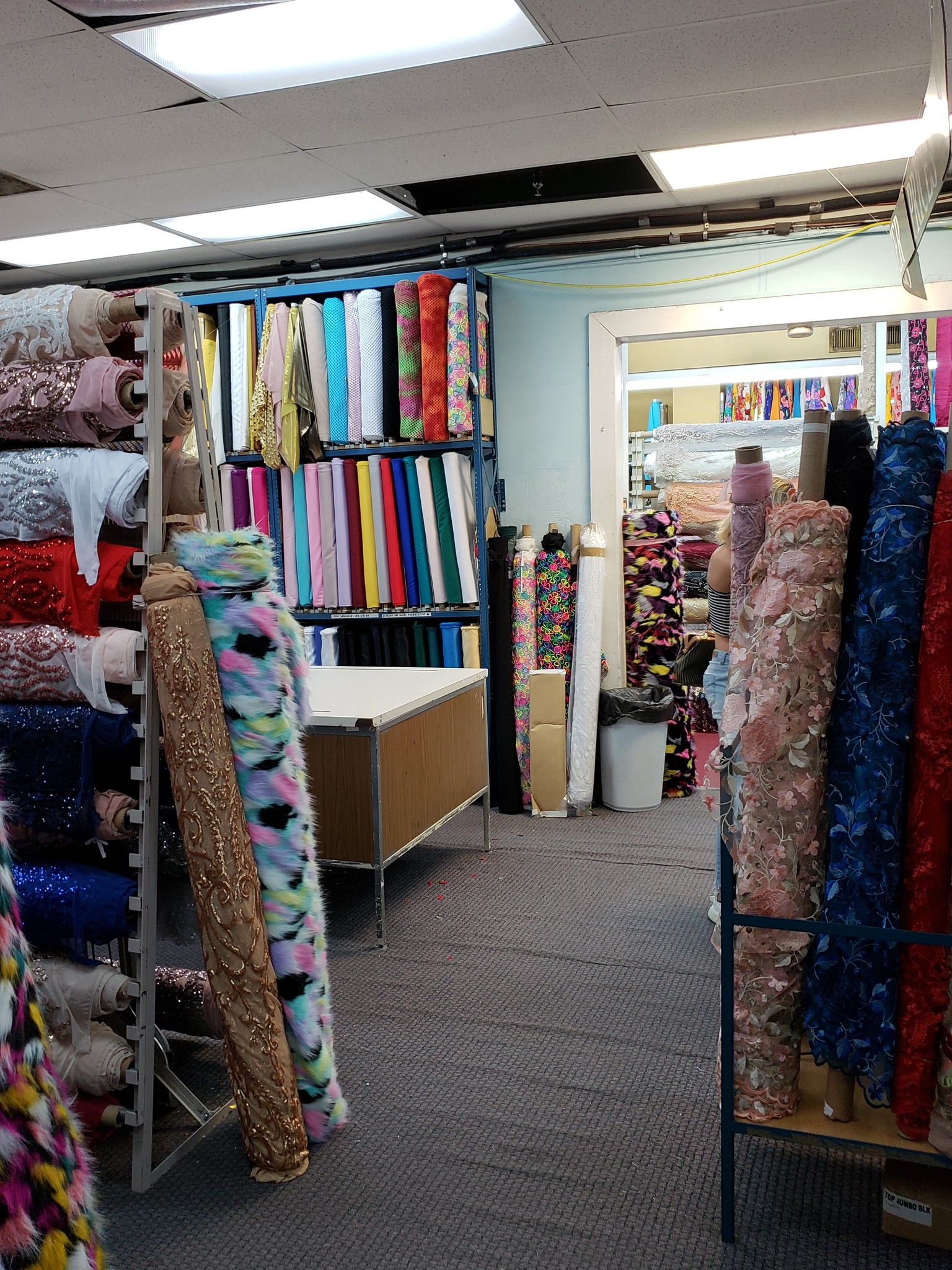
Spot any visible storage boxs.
[883,1160,952,1250]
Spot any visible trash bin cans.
[599,686,675,810]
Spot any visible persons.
[703,473,794,926]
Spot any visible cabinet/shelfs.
[0,268,498,1192]
